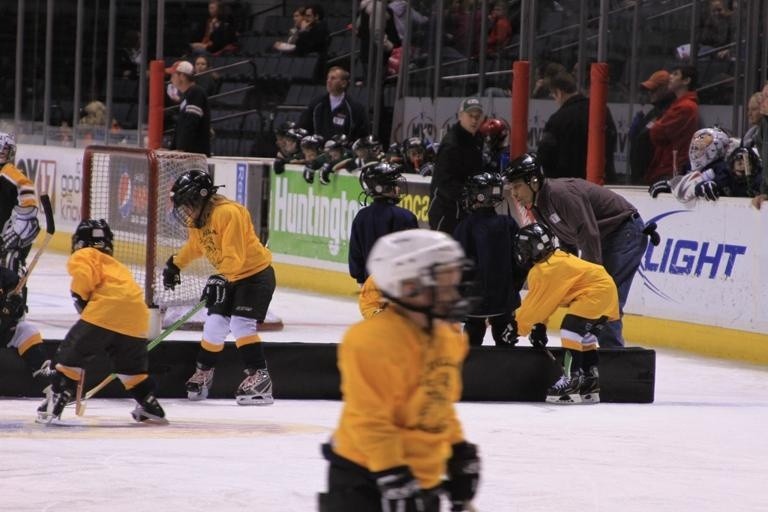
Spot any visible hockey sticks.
[14,194,53,296]
[538,340,572,379]
[66,296,207,418]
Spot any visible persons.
[350,162,419,278]
[318,228,485,511]
[0,131,55,395]
[357,277,387,319]
[503,223,619,396]
[507,153,647,350]
[46,1,768,217]
[40,219,165,420]
[164,170,276,394]
[447,171,521,346]
[426,98,492,235]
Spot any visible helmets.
[358,160,407,203]
[0,131,19,165]
[688,126,732,174]
[460,171,505,212]
[479,117,510,143]
[504,150,543,183]
[513,223,555,264]
[168,168,215,230]
[70,218,114,256]
[274,122,426,166]
[366,228,474,313]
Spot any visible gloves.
[162,252,182,292]
[199,274,228,310]
[699,180,720,202]
[444,442,480,503]
[528,322,549,347]
[648,178,673,198]
[374,463,440,511]
[501,318,520,346]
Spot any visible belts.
[613,212,641,232]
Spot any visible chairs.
[0,1,768,169]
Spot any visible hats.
[639,69,671,91]
[164,60,195,77]
[457,97,483,114]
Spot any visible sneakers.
[33,360,61,391]
[547,372,601,395]
[130,392,165,422]
[37,391,70,417]
[235,363,272,396]
[183,365,214,393]
